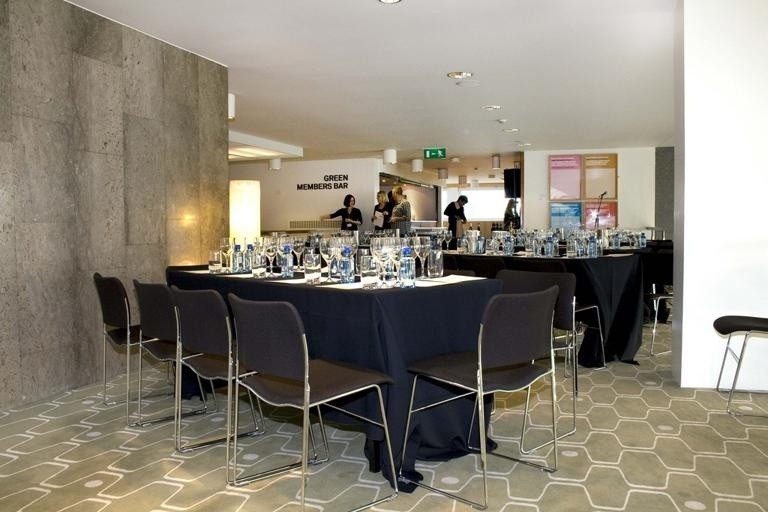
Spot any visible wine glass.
[209,227,453,290]
[455,228,648,258]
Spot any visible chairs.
[92,273,208,426]
[462,268,579,457]
[134,276,260,449]
[400,284,561,509]
[228,291,398,512]
[712,311,768,418]
[170,285,317,485]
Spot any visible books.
[373,210,385,229]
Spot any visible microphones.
[601,191,607,197]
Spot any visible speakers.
[504,169,522,198]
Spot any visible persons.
[504,199,520,232]
[386,191,398,206]
[319,194,363,247]
[371,190,393,230]
[443,195,468,236]
[390,185,411,237]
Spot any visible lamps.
[229,179,261,251]
[268,158,280,171]
[383,149,397,165]
[458,175,466,184]
[491,155,500,169]
[513,161,520,169]
[437,167,447,180]
[228,92,236,121]
[411,159,423,172]
